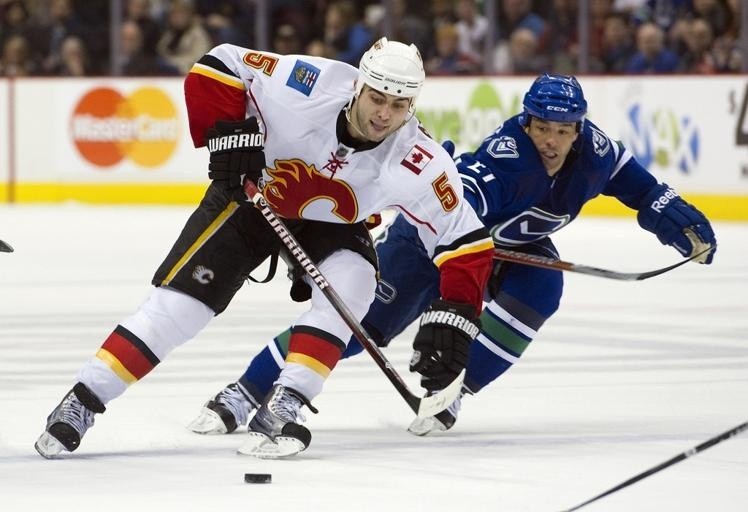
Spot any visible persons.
[200,70,718,436]
[44,36,499,455]
[3,1,253,77]
[275,1,487,74]
[495,0,747,75]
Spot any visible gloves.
[637,184,716,264]
[207,116,267,208]
[409,301,482,390]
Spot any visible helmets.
[354,36,425,113]
[522,73,587,134]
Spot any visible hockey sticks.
[241,178,467,419]
[493,244,717,282]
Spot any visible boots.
[206,382,258,434]
[426,383,473,431]
[247,384,318,452]
[46,382,105,451]
